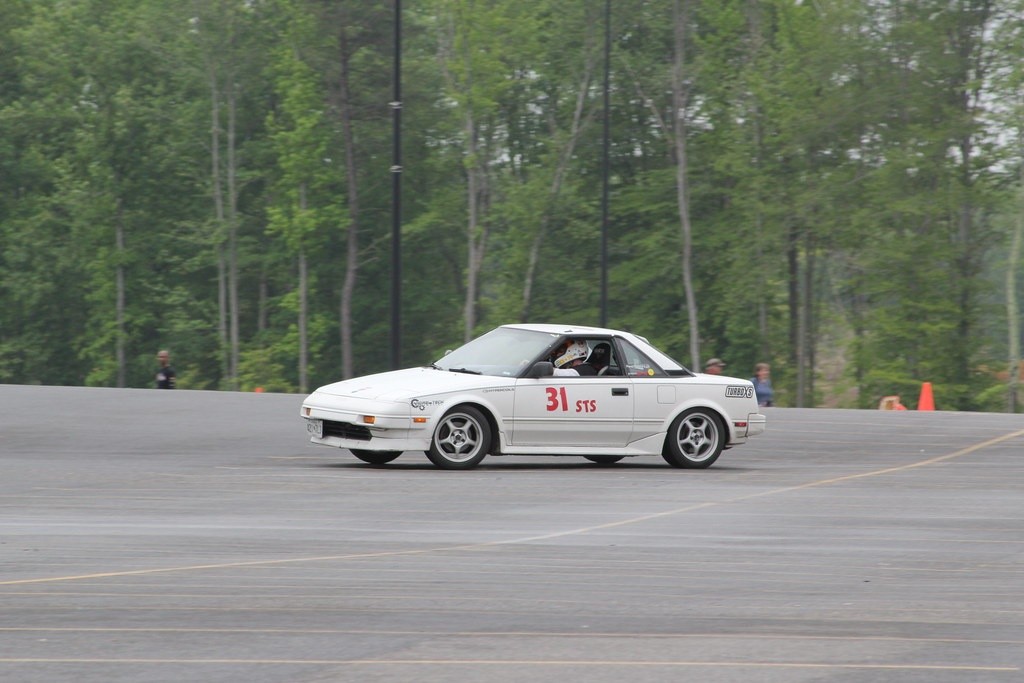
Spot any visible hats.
[707,358,726,368]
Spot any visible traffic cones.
[254,387,265,394]
[915,381,937,411]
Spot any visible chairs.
[583,343,611,376]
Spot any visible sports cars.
[298,323,767,471]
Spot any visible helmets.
[554,337,592,368]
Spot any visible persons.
[704,358,725,375]
[156,350,176,389]
[520,339,596,376]
[749,363,772,407]
